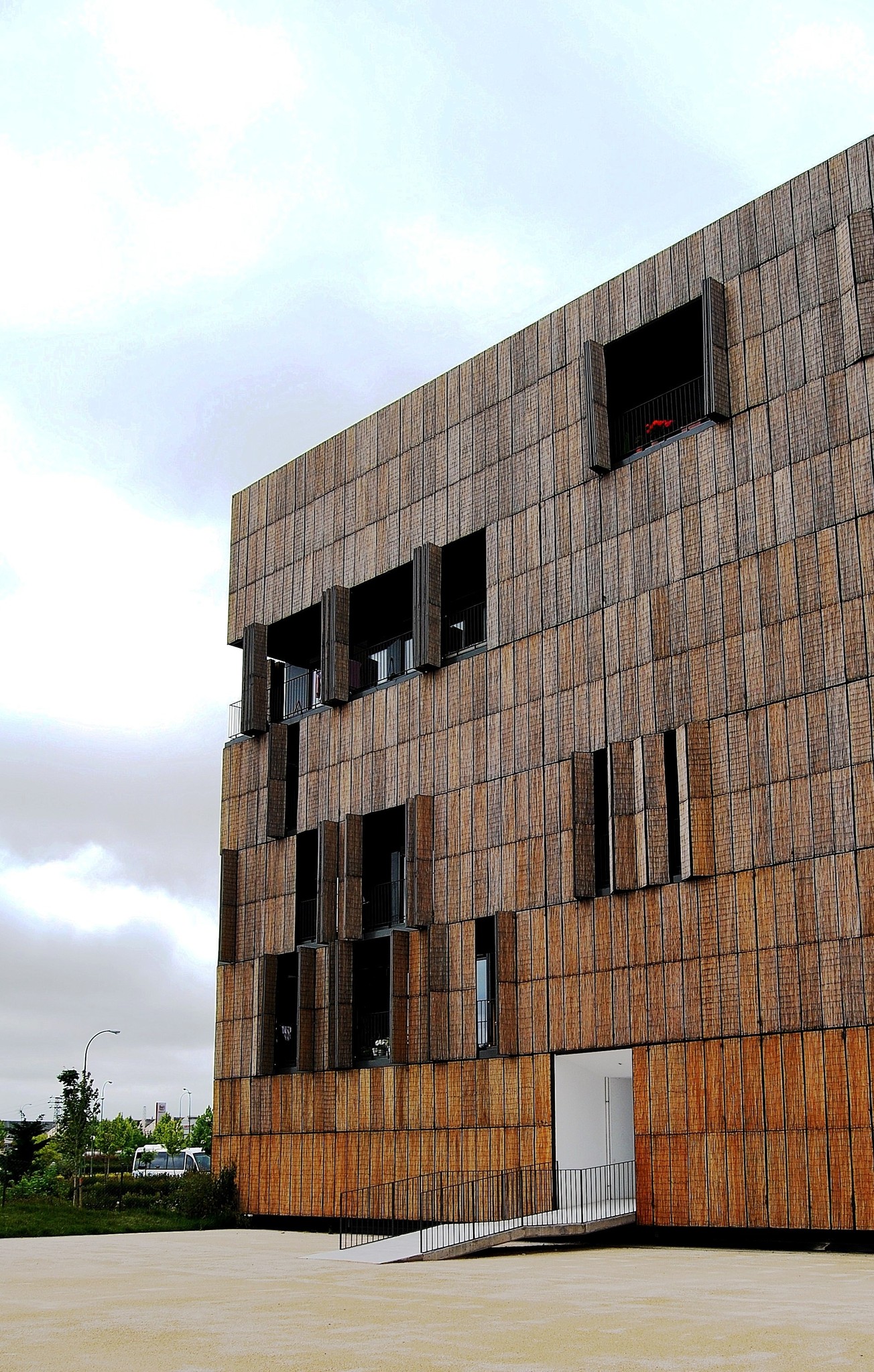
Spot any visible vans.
[130,1143,211,1178]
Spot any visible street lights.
[20,1104,32,1120]
[83,1028,121,1077]
[99,1080,113,1155]
[90,1096,105,1175]
[183,1088,191,1135]
[179,1092,192,1123]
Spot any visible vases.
[378,1049,384,1056]
[652,441,658,446]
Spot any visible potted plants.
[634,435,645,453]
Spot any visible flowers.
[646,419,674,441]
[375,1039,389,1049]
